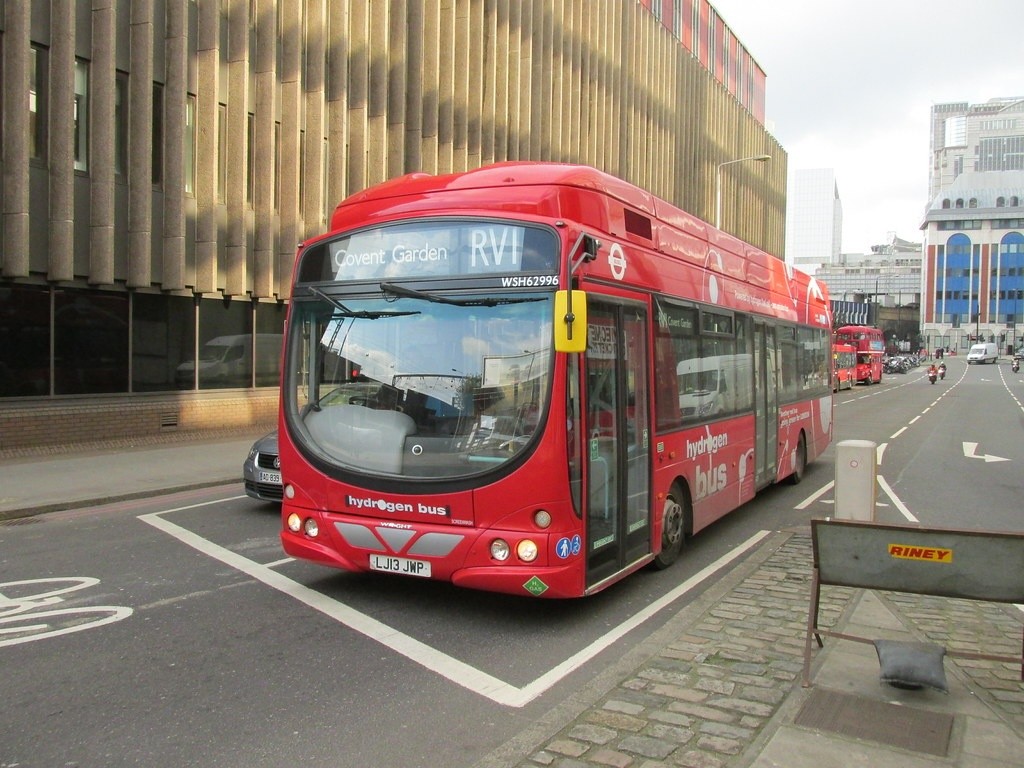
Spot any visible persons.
[938,362,946,370]
[1011,357,1019,365]
[936,347,944,359]
[928,364,938,374]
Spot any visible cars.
[243,428,283,504]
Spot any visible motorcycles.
[927,370,938,384]
[938,367,945,380]
[1011,357,1020,374]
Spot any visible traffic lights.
[351,362,361,380]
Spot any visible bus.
[832,343,858,394]
[273,162,838,610]
[837,324,886,386]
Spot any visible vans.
[675,352,773,423]
[176,332,285,391]
[966,342,999,365]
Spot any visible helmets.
[941,362,944,365]
[931,364,935,368]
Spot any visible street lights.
[715,153,771,234]
[452,368,467,446]
[872,275,900,327]
[509,350,535,440]
[1012,289,1020,357]
